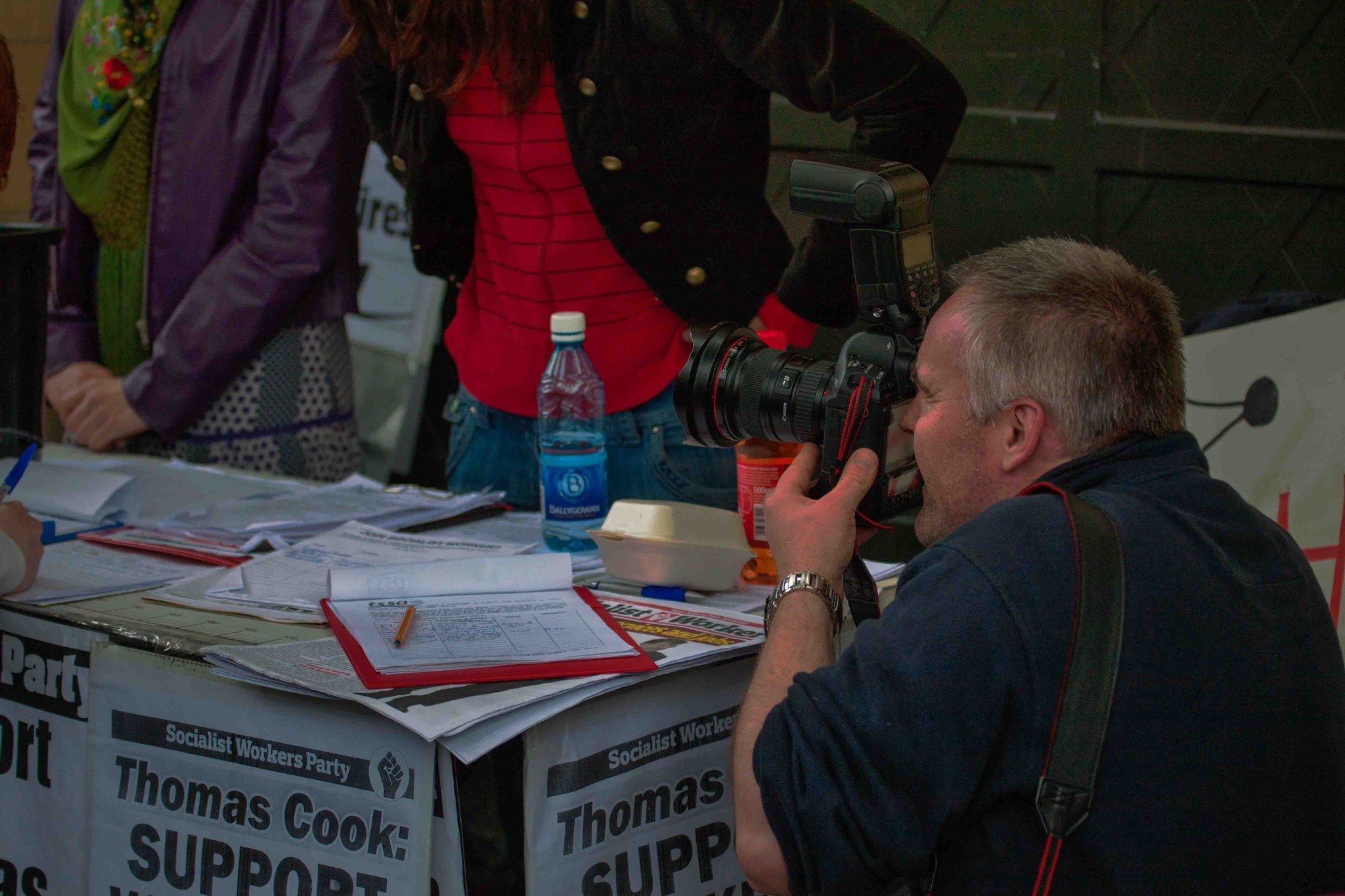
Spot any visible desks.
[0,439,899,896]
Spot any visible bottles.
[538,311,610,551]
[734,326,802,586]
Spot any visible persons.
[733,240,1345,896]
[329,0,970,512]
[0,500,44,596]
[25,0,369,486]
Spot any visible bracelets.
[764,571,842,640]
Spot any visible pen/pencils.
[0,443,38,500]
[394,605,416,648]
[397,503,514,533]
[581,581,704,601]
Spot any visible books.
[320,553,659,689]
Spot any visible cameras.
[670,149,944,530]
